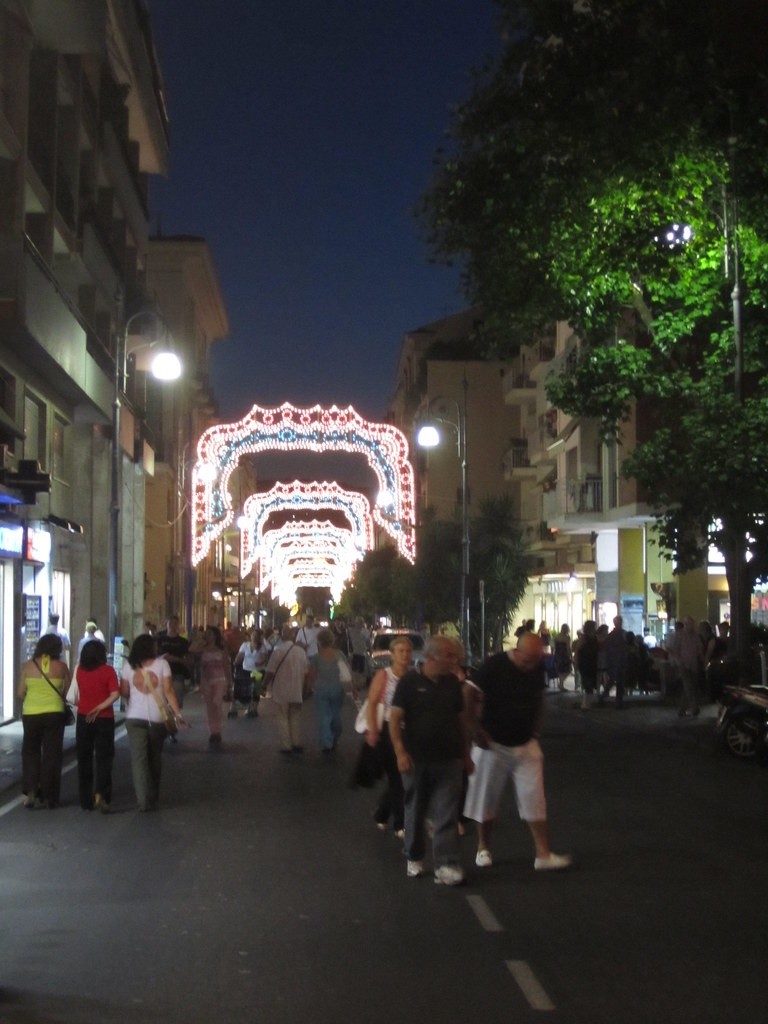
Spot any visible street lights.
[107,307,182,651]
[418,395,476,668]
[654,222,748,684]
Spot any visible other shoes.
[373,813,386,831]
[476,851,491,867]
[533,852,570,870]
[245,710,258,717]
[394,829,406,838]
[228,711,237,717]
[95,794,109,815]
[209,734,224,745]
[24,791,34,808]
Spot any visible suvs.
[369,629,426,678]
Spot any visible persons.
[41,612,384,753]
[366,635,412,838]
[461,632,574,873]
[514,615,731,719]
[388,636,465,886]
[16,633,72,807]
[119,633,191,813]
[75,640,120,814]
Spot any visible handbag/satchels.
[336,653,352,682]
[161,706,177,733]
[354,669,387,734]
[260,678,274,699]
[63,705,75,726]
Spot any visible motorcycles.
[710,681,767,758]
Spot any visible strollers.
[231,664,266,717]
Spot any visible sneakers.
[433,865,462,885]
[408,861,424,876]
